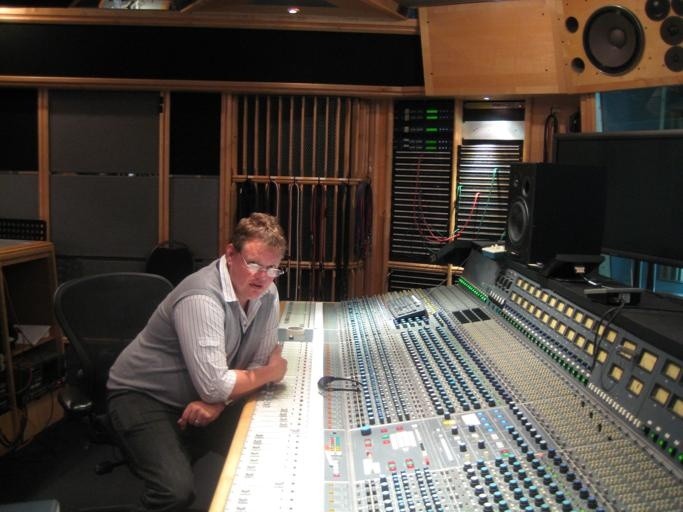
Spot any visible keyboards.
[386,292,426,321]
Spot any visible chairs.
[46,270,178,482]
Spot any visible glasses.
[238,252,284,278]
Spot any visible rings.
[195,420,201,425]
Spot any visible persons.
[105,211,288,511]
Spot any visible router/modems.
[583,288,642,306]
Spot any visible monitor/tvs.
[551,129,683,290]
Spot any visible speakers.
[556,0,683,95]
[504,162,609,265]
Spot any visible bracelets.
[224,397,234,408]
